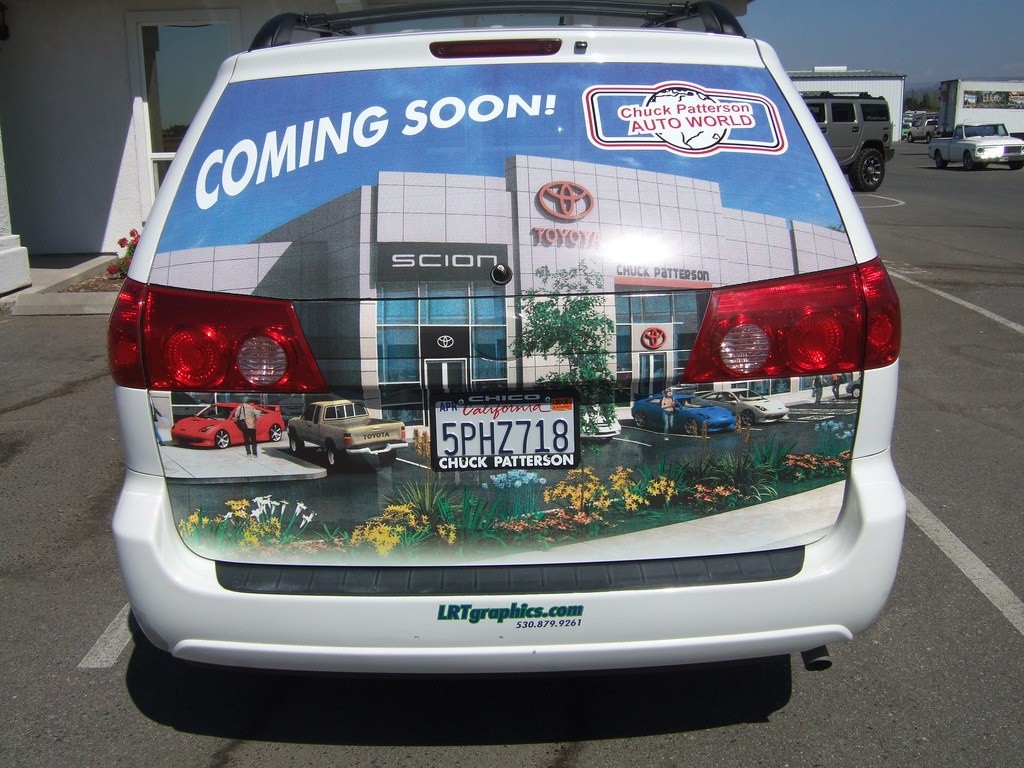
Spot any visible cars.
[902,124,912,141]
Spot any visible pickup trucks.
[907,118,942,143]
[928,120,1024,171]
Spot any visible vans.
[105,0,911,676]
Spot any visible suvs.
[796,89,897,192]
